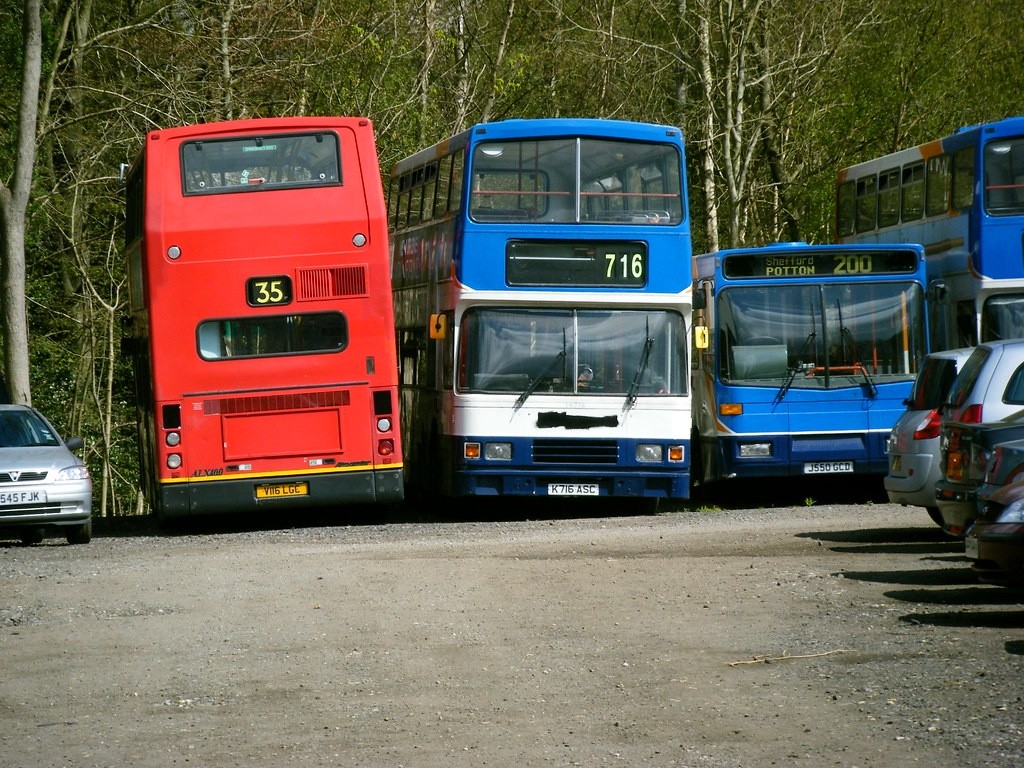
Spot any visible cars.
[966,478,1023,587]
[976,440,1024,518]
[0,404,94,544]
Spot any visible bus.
[123,115,405,525]
[388,119,692,523]
[836,115,1024,352]
[691,242,932,502]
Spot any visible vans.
[883,339,1023,538]
